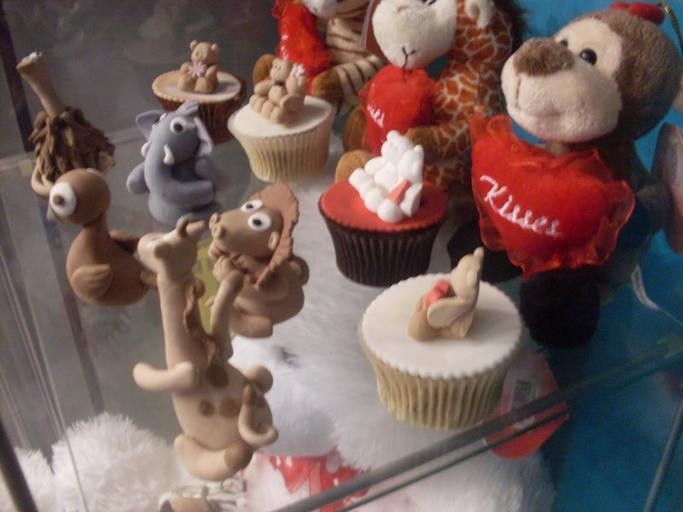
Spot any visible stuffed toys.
[252,0,523,214]
[447,1,683,352]
